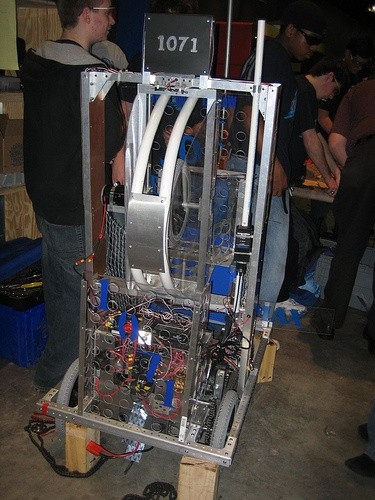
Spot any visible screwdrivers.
[12,282,42,289]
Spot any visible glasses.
[295,26,322,46]
[78,7,116,18]
[350,53,368,66]
[331,74,341,96]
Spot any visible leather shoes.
[362,324,375,353]
[311,313,335,340]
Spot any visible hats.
[287,3,331,37]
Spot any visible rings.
[330,191,335,194]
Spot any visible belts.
[354,133,375,147]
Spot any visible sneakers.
[260,297,308,317]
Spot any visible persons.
[225,10,375,353]
[345,398,375,478]
[20,0,171,395]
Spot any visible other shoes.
[345,453,375,476]
[31,386,78,404]
[359,423,370,441]
